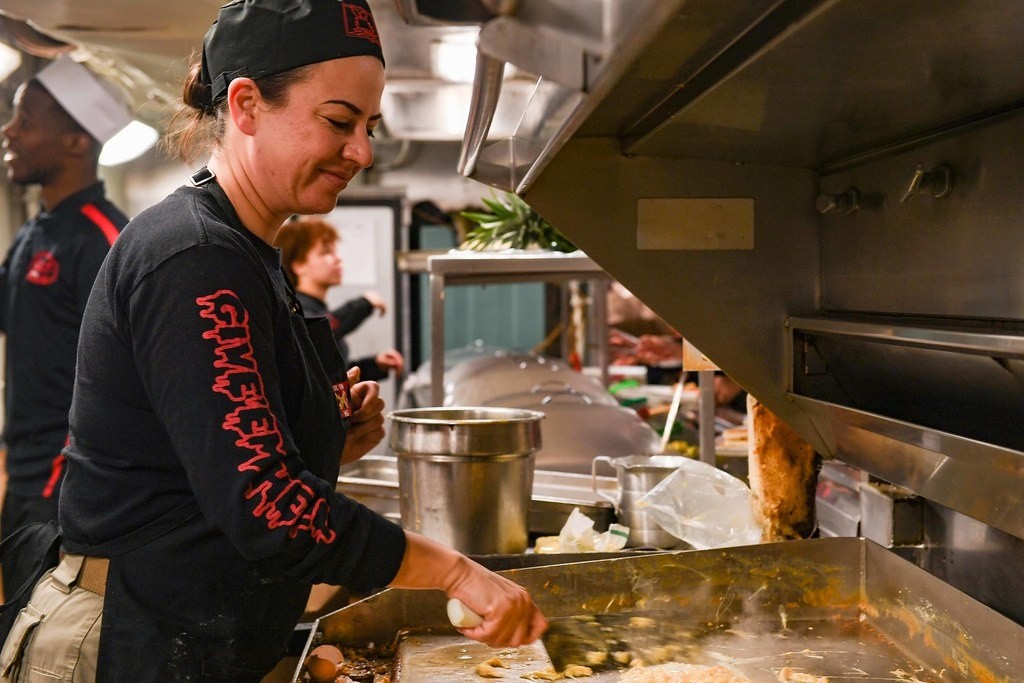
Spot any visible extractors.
[456,0,1024,540]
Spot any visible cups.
[591,455,693,549]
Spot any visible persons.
[0,52,131,647]
[54,0,549,683]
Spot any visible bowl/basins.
[386,407,547,554]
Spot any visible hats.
[35,51,135,145]
[200,0,386,105]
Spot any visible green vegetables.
[459,186,579,253]
